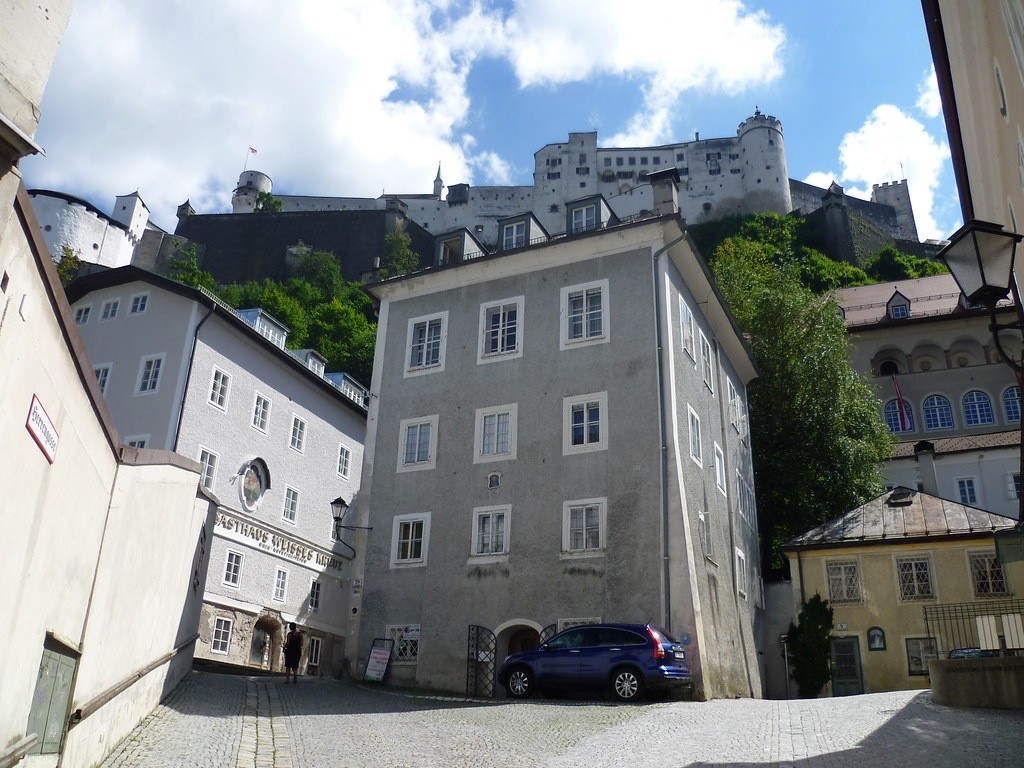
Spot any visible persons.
[283,622,303,684]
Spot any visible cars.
[947,647,987,659]
[496,622,694,703]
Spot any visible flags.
[894,376,911,431]
